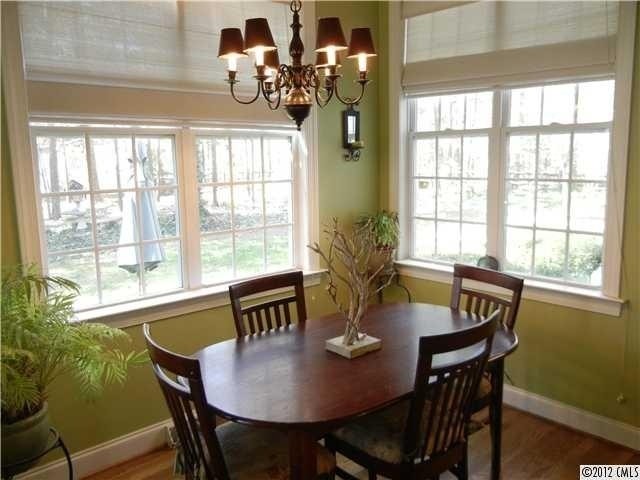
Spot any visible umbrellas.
[117,142,163,297]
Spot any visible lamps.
[216,1,378,134]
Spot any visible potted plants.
[0,261,150,471]
[356,209,400,275]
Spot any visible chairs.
[142,262,522,479]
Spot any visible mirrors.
[342,102,364,162]
